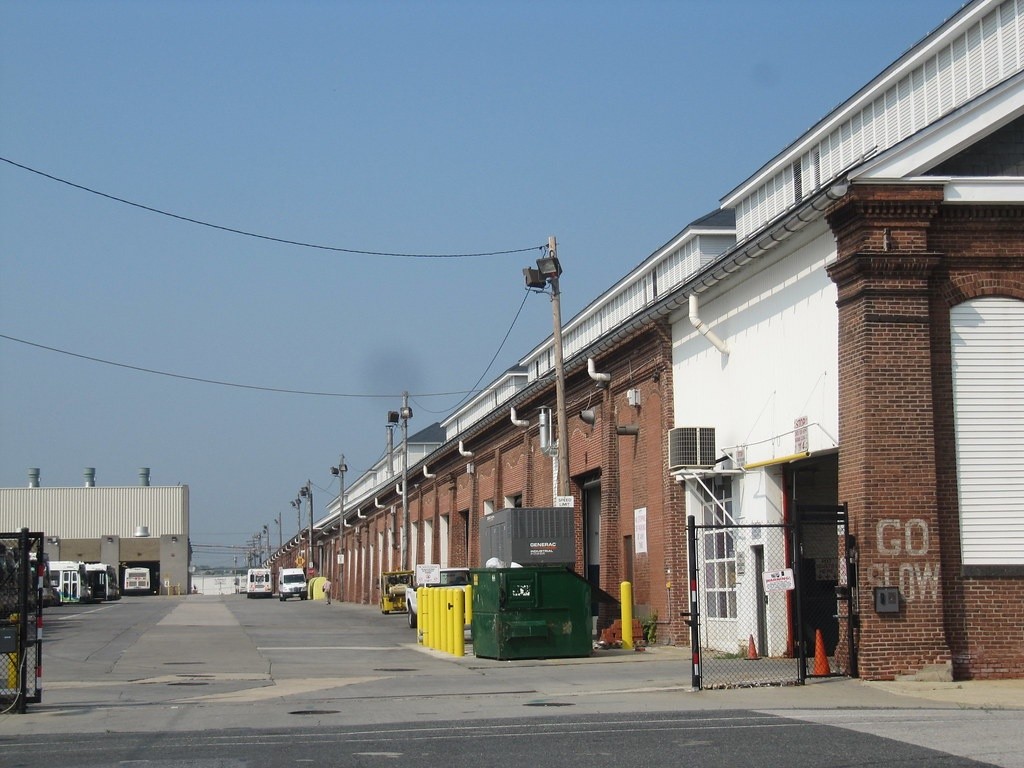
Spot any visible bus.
[48,560,89,604]
[124,568,151,593]
[248,567,273,598]
[25,550,52,601]
[84,564,122,602]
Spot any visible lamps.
[108,538,113,542]
[172,537,177,541]
[47,539,52,543]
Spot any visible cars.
[405,567,471,630]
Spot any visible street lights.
[263,478,314,575]
[522,235,571,497]
[331,454,348,601]
[387,391,412,569]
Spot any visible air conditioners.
[667,426,716,469]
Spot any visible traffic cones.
[809,627,835,678]
[744,633,761,661]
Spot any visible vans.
[279,567,309,601]
